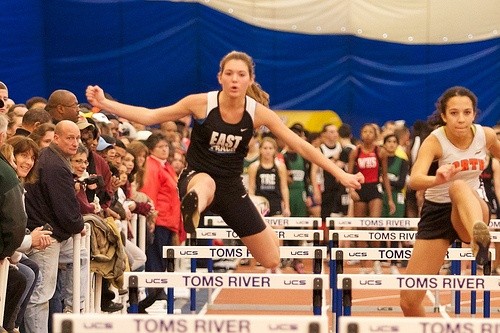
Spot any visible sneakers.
[391,266,400,275]
[373,261,383,274]
[181,190,199,233]
[472,220,492,264]
[106,302,123,313]
[252,197,269,215]
[347,260,360,265]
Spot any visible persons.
[0,83,500,333]
[86,51,365,269]
[400,86,500,317]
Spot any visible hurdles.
[53,216,500,333]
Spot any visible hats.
[93,113,114,128]
[97,137,114,151]
[76,112,95,130]
[136,130,152,141]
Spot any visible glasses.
[63,104,81,108]
[72,159,89,166]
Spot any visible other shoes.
[118,290,128,294]
[156,295,176,300]
